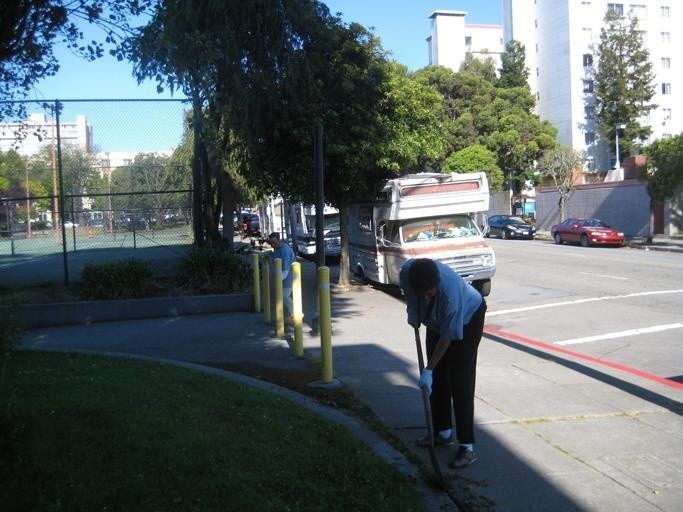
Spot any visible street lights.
[41,99,70,292]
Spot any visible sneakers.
[417,429,454,447]
[452,446,477,467]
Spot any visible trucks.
[347,170,495,303]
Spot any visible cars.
[0,212,192,234]
[480,214,538,239]
[550,216,627,249]
[220,207,261,237]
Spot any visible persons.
[400,257,486,468]
[449,220,469,237]
[265,232,304,324]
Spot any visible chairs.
[404,224,469,239]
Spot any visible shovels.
[415,328,445,487]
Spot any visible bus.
[256,181,346,259]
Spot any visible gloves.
[408,309,421,326]
[418,368,433,394]
